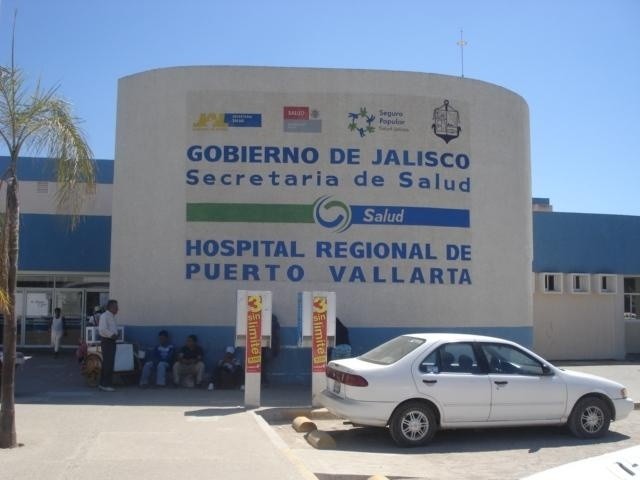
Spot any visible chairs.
[445,351,473,372]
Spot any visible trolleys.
[83,343,140,388]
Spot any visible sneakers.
[98,383,117,392]
[207,382,215,391]
[239,384,245,391]
[137,381,203,390]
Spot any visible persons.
[137,330,207,392]
[329,317,354,362]
[262,314,282,387]
[85,299,120,392]
[208,345,242,392]
[48,307,67,354]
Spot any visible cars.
[320,332,634,447]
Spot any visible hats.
[224,346,235,355]
